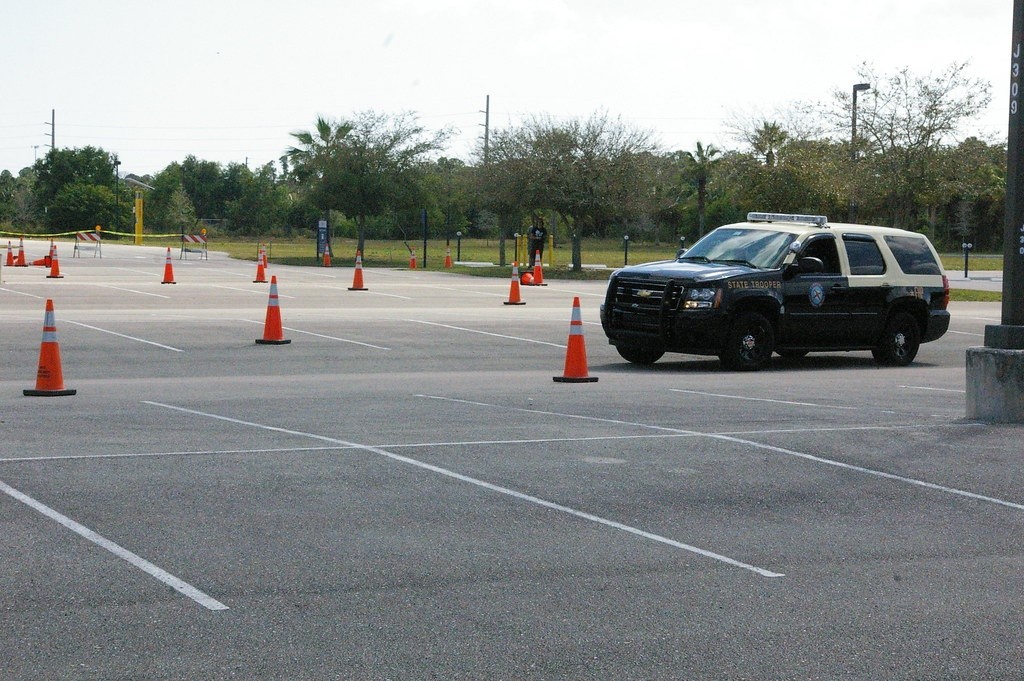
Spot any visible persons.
[528,219,546,268]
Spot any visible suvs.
[597,211,952,372]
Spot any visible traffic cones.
[261,243,269,269]
[519,271,534,284]
[442,247,453,269]
[321,242,333,267]
[531,250,548,286]
[160,246,177,285]
[252,249,268,284]
[27,256,51,268]
[14,236,29,266]
[255,275,292,345]
[46,237,54,269]
[503,261,526,305]
[3,240,15,266]
[408,247,418,270]
[13,255,19,264]
[550,296,601,383]
[348,248,369,290]
[46,246,64,279]
[23,299,77,396]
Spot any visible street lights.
[850,82,870,223]
